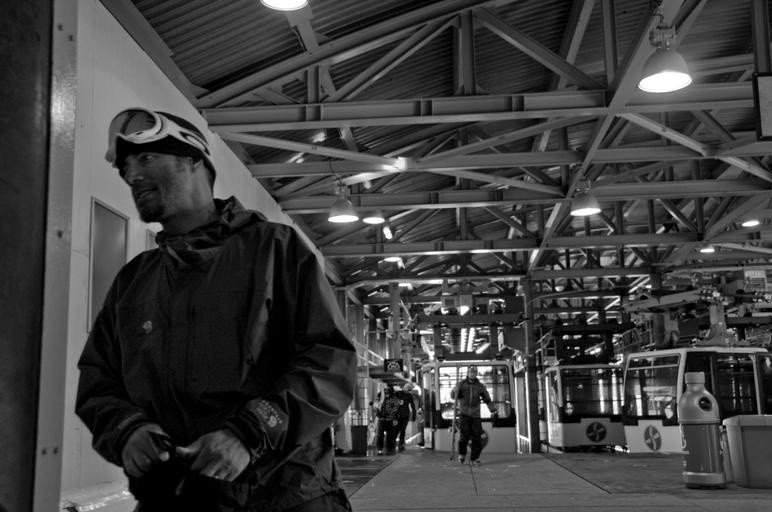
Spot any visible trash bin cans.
[351,425,368,456]
[679,371,727,489]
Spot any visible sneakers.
[471,459,480,465]
[458,455,465,463]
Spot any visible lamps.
[328,177,359,224]
[637,9,692,94]
[570,177,601,217]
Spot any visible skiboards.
[450,362,462,459]
[366,383,385,458]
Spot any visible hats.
[115,112,215,190]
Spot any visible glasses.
[106,107,216,171]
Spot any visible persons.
[451,363,499,467]
[74,106,359,512]
[395,383,417,451]
[372,385,399,455]
